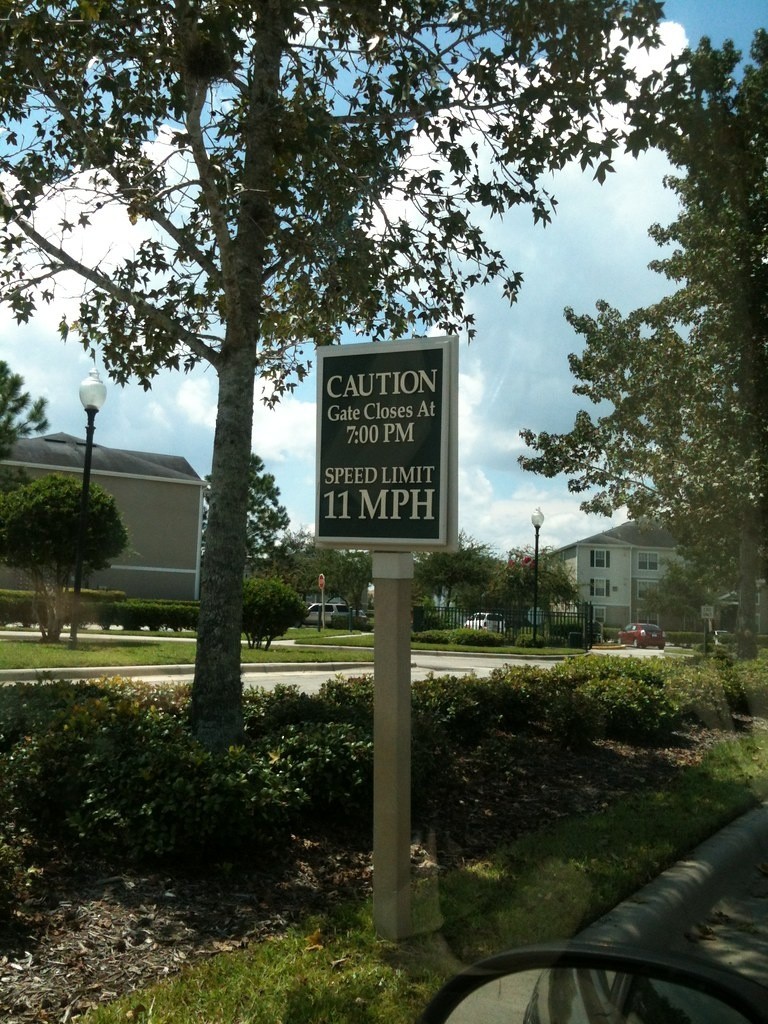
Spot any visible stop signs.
[318,574,325,589]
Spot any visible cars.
[351,610,368,624]
[618,622,665,650]
[712,630,728,642]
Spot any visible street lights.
[67,369,108,644]
[532,507,544,648]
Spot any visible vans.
[464,614,506,635]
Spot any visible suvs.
[295,602,351,627]
